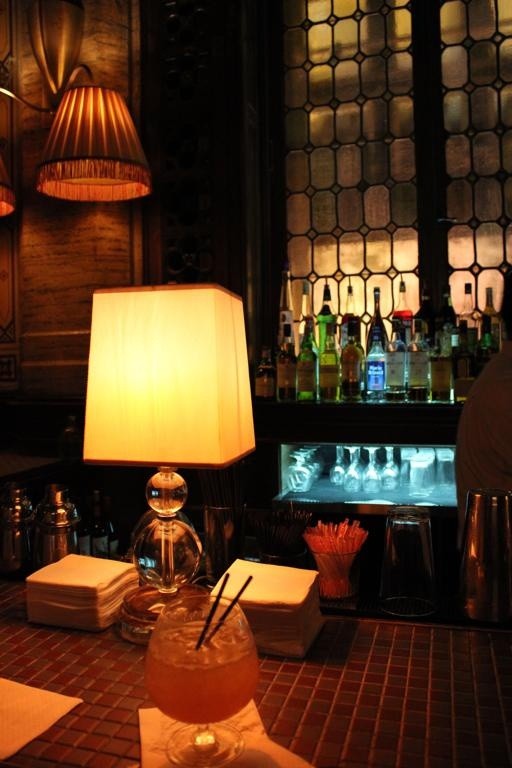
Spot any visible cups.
[376,506,440,617]
[309,551,360,601]
[202,505,246,585]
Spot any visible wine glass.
[144,594,261,768]
[399,448,454,498]
[286,446,400,492]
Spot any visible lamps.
[1,62,153,203]
[80,282,257,646]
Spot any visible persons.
[453,268,512,549]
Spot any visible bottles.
[457,487,512,622]
[255,281,501,402]
[0,481,119,576]
